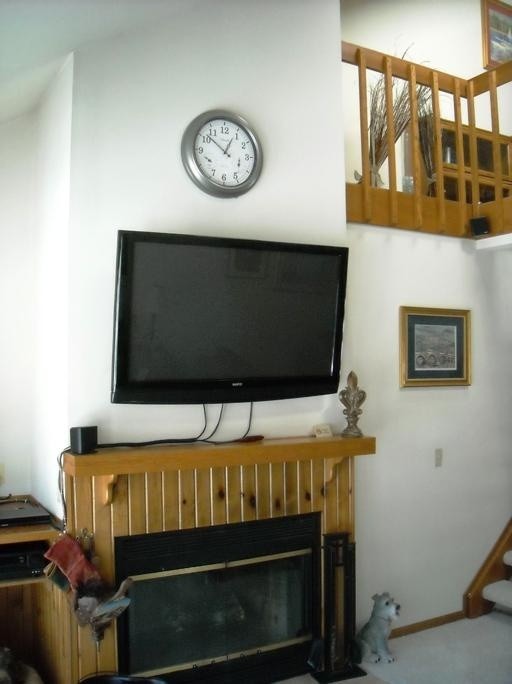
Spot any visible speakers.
[69,426,98,455]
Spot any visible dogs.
[356,590,401,665]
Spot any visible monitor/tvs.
[110,229,350,405]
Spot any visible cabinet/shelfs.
[404,114,512,202]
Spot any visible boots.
[43,533,133,633]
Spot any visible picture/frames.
[481,0,512,70]
[400,306,471,387]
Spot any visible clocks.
[181,110,263,197]
